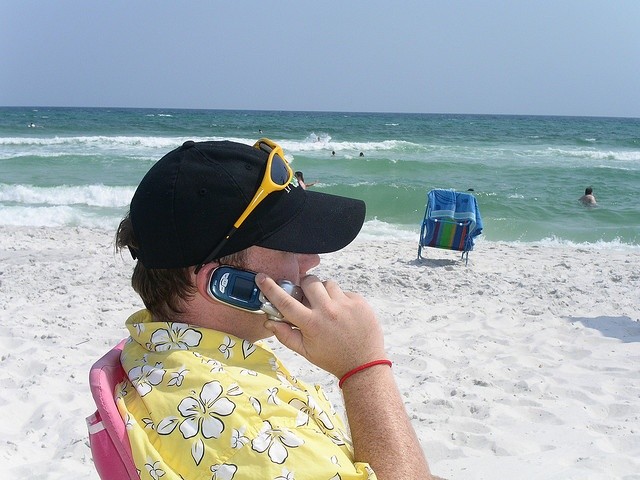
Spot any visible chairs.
[417,189,484,268]
[89,337,139,480]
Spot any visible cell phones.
[206,265,311,328]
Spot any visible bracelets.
[333,356,392,396]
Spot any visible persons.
[359,151,365,159]
[577,184,598,212]
[330,150,336,159]
[88,135,433,480]
[294,172,318,192]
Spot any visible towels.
[426,190,482,239]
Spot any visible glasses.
[194,138,293,274]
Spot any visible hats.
[128,140,366,269]
